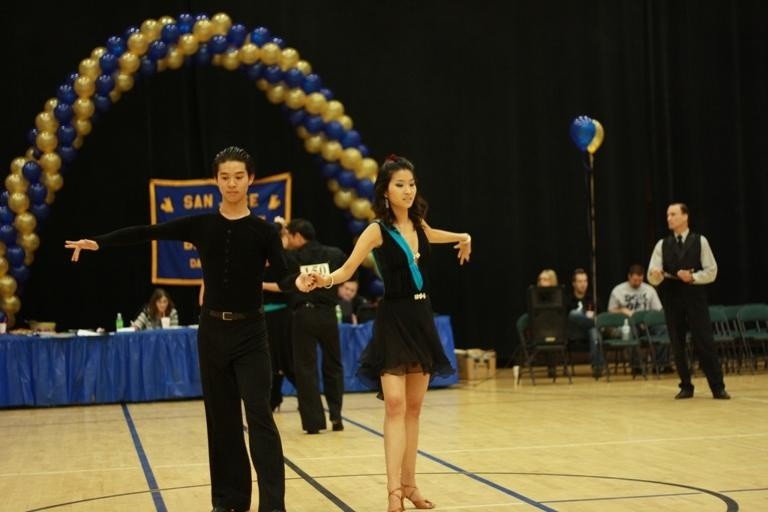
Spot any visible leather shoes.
[307,413,343,434]
[713,389,729,398]
[675,388,693,398]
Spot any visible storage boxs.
[455,349,497,381]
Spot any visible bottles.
[621,318,632,341]
[115,313,124,333]
[334,305,343,324]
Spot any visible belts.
[201,306,264,321]
[296,303,335,309]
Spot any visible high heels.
[271,393,282,411]
[388,486,404,511]
[401,481,435,509]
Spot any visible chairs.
[516,303,768,386]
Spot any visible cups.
[161,317,170,331]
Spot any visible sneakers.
[632,366,674,375]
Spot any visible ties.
[678,234,682,247]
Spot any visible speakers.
[525,286,567,346]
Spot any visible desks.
[0,316,458,409]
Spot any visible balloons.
[570,116,596,154]
[258,46,368,168]
[320,149,385,297]
[2,98,63,333]
[588,120,606,157]
[54,36,140,166]
[120,13,272,77]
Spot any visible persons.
[606,265,678,378]
[553,266,610,377]
[260,219,381,436]
[647,202,735,400]
[335,280,372,325]
[63,147,322,511]
[132,290,178,331]
[301,152,475,512]
[522,268,563,379]
